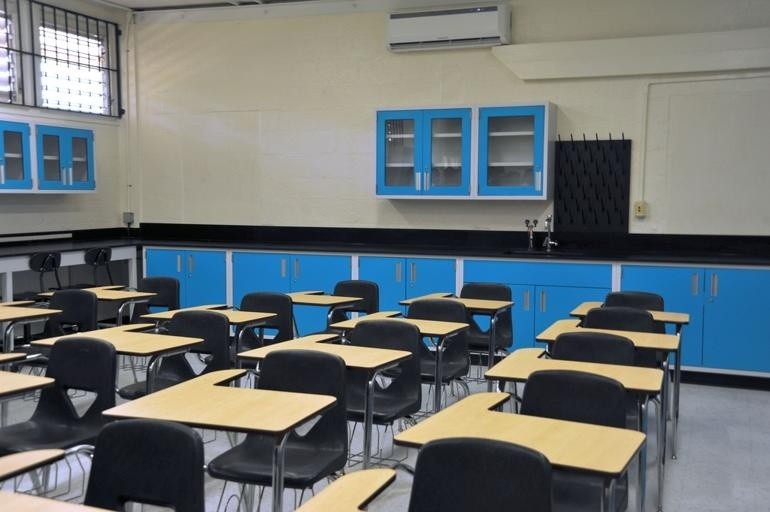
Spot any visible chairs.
[13,252,63,300]
[68,247,116,288]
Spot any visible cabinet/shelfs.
[375,106,474,200]
[616,262,770,378]
[142,246,229,315]
[460,258,616,357]
[354,255,461,351]
[229,250,354,339]
[33,123,95,193]
[474,101,556,201]
[0,117,35,194]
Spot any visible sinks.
[501,250,583,259]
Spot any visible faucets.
[526,223,537,250]
[542,218,559,252]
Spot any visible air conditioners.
[385,2,512,52]
